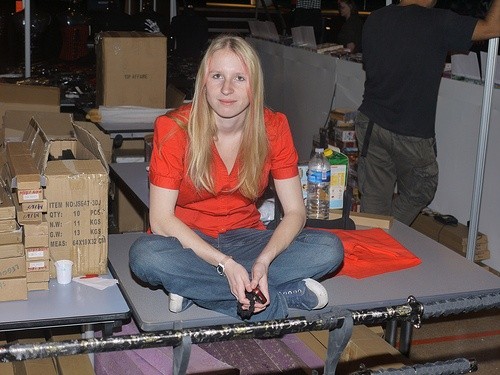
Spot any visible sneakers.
[275,278,328,311]
[168,293,194,313]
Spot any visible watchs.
[217,255,233,276]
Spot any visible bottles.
[307,148,331,219]
[324,148,349,209]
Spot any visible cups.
[54,260,73,285]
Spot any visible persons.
[337,0,365,47]
[355,0,500,227]
[295,0,322,46]
[128,34,345,322]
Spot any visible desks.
[0,266,131,375]
[107,215,500,375]
[108,160,151,232]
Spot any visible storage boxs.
[411,212,489,271]
[0,323,420,375]
[308,107,394,230]
[93,31,167,108]
[0,82,115,301]
